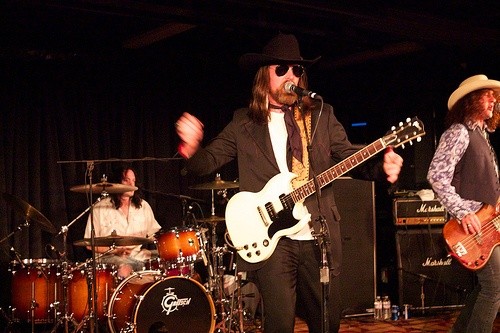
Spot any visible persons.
[83,162,161,278]
[172,32,404,333]
[425,74,500,333]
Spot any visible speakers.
[302,177,378,317]
[394,227,475,316]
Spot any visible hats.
[239,34,321,63]
[448,74,500,110]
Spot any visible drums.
[154,224,203,265]
[66,263,117,320]
[108,270,217,333]
[9,259,64,323]
[146,258,194,277]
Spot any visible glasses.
[268,64,304,78]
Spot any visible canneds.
[402,304,411,320]
[392,305,400,321]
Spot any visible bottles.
[374,296,392,321]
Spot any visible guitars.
[224,115,428,264]
[443,203,500,270]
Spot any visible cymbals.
[69,182,138,193]
[73,235,152,247]
[187,179,240,189]
[195,215,224,222]
[1,192,57,234]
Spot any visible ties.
[268,102,303,172]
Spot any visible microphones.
[284,81,322,100]
[183,205,192,225]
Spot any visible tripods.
[215,270,263,333]
[52,203,110,333]
